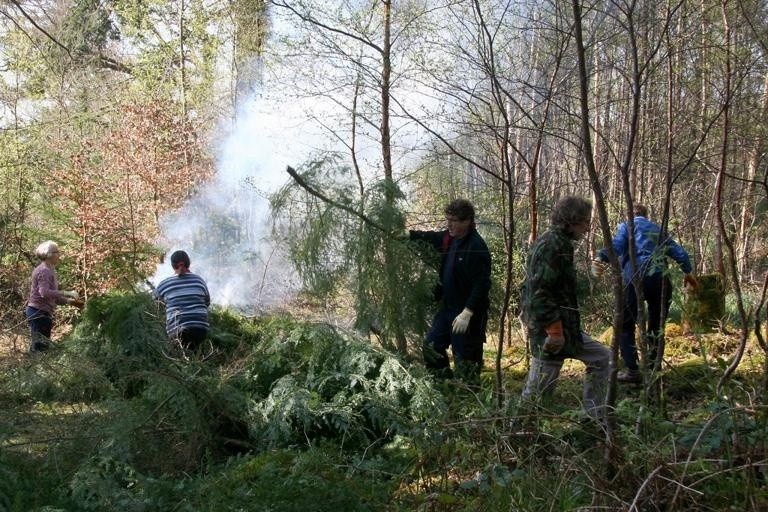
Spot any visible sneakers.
[615,369,643,384]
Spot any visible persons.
[22,236,82,356]
[149,249,214,353]
[394,196,494,392]
[589,203,701,388]
[518,192,614,418]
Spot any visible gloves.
[63,289,79,301]
[66,298,83,308]
[392,228,410,239]
[452,307,473,335]
[591,257,604,277]
[543,320,564,354]
[683,272,699,290]
